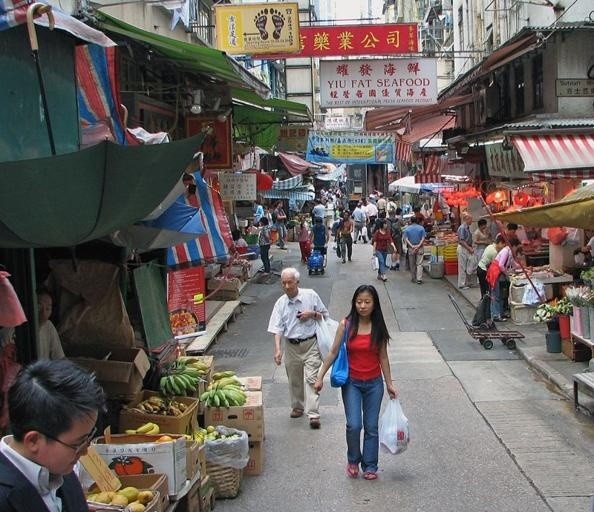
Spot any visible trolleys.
[471,287,495,329]
[305,241,329,276]
[447,293,524,350]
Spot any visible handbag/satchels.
[336,241,341,259]
[371,254,379,271]
[316,317,340,362]
[330,341,349,388]
[466,254,479,276]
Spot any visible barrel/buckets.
[545,331,562,354]
[428,260,445,279]
[545,320,560,331]
[247,244,259,260]
[236,245,249,254]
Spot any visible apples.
[158,436,171,442]
[113,456,143,476]
[87,486,153,512]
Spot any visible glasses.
[37,426,98,454]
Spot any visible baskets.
[440,241,460,260]
[444,258,460,276]
[205,431,249,499]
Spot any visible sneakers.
[346,461,359,478]
[310,417,321,427]
[493,316,507,322]
[289,407,304,418]
[354,231,372,244]
[377,273,388,281]
[411,278,424,284]
[363,471,378,480]
[259,272,270,277]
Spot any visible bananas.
[137,357,247,416]
[125,421,160,435]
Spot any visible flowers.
[533,303,559,322]
[555,297,574,316]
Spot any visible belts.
[287,333,316,345]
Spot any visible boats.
[311,149,329,156]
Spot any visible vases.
[545,318,559,330]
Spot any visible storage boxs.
[207,260,253,301]
[76,343,267,512]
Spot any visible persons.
[456,214,523,328]
[267,267,330,429]
[298,186,354,263]
[252,201,288,274]
[0,357,108,512]
[351,191,433,283]
[314,285,395,481]
[37,285,66,360]
[582,230,594,266]
